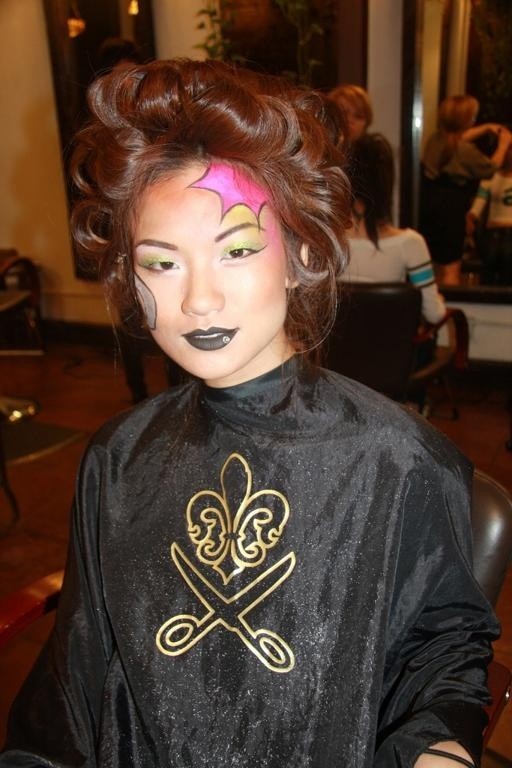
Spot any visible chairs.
[321,282,468,419]
[420,179,491,272]
[0,468,512,768]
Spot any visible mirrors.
[218,0,368,95]
[43,0,157,283]
[399,0,511,305]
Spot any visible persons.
[0,58,502,768]
[328,84,456,350]
[420,95,512,284]
